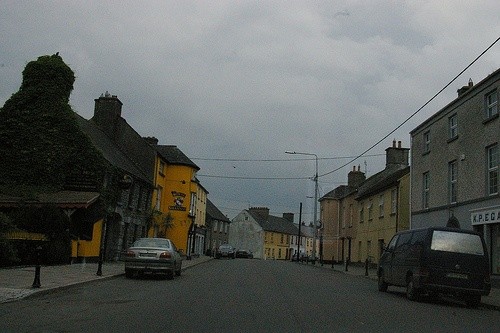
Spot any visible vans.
[375,226,492,307]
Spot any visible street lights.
[284,151,319,264]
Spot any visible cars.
[124,237,183,280]
[235,248,254,259]
[215,244,236,259]
